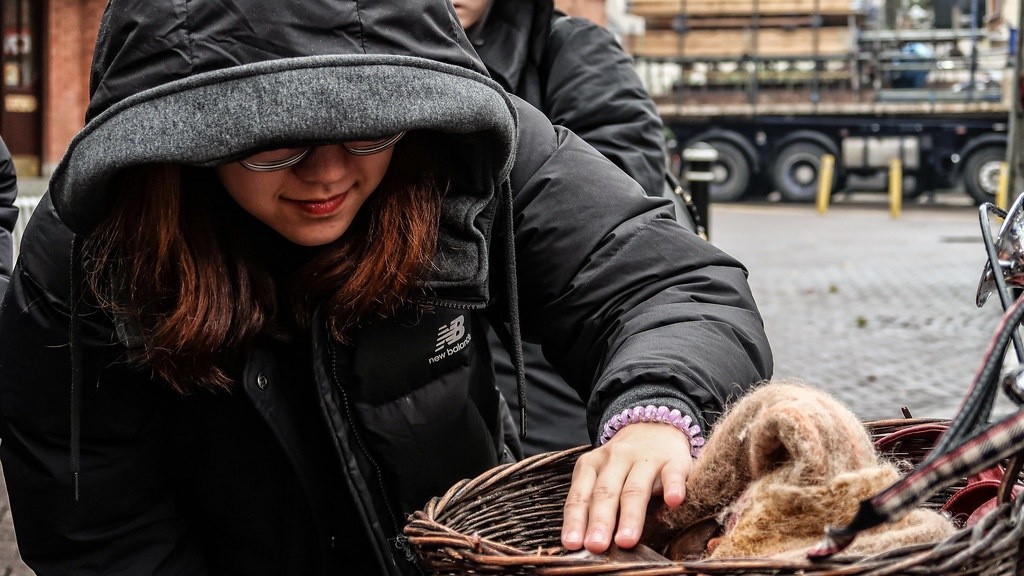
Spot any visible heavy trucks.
[554,0,1024,211]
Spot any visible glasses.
[239,129,408,172]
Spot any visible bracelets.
[599,404,704,463]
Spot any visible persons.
[450,0,667,201]
[0,0,774,576]
[0,136,20,305]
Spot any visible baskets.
[402,417,1024,576]
